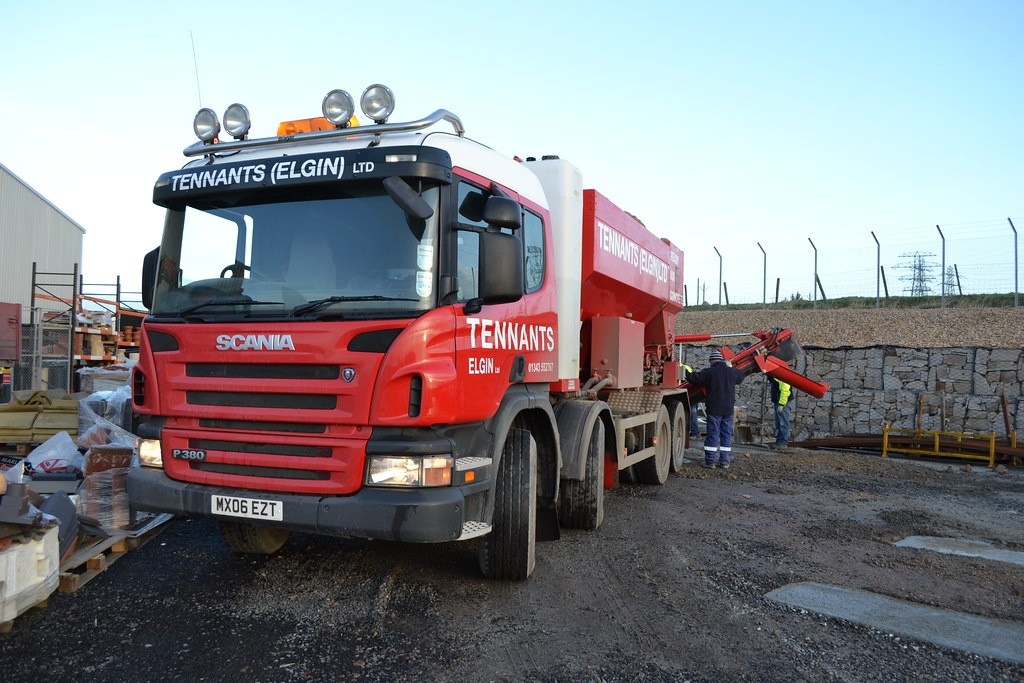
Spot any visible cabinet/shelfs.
[26,261,150,394]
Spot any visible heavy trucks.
[123,84,693,581]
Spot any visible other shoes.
[720,463,729,469]
[701,462,716,468]
[689,434,700,439]
[770,443,788,450]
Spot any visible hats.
[709,349,723,362]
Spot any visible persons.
[685,348,746,469]
[766,374,794,449]
[676,363,700,438]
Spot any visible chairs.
[282,234,335,288]
